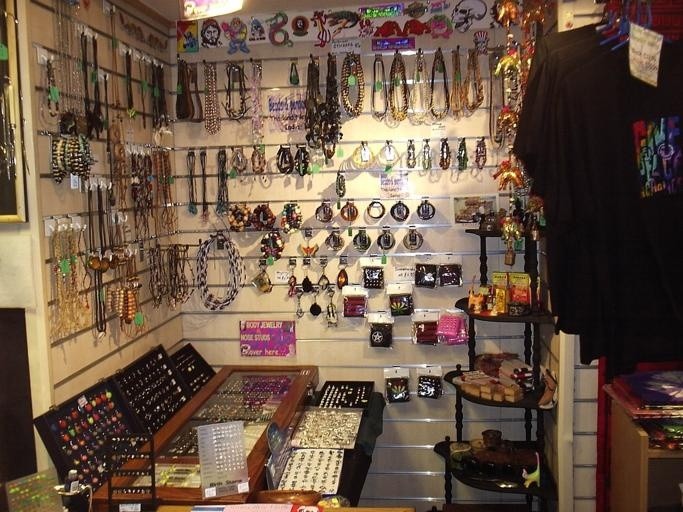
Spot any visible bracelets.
[228,137,488,261]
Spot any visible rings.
[51,353,365,493]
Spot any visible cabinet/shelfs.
[432,229,556,512]
[610,403,683,512]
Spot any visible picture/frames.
[450,193,500,229]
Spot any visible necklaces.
[0,0,263,343]
[304,45,484,158]
